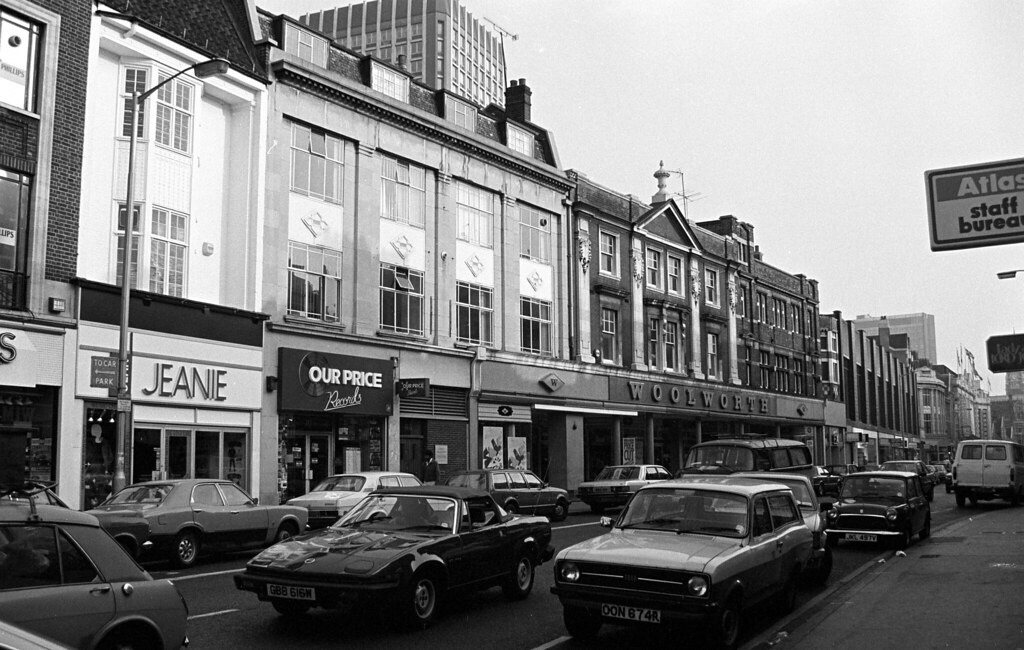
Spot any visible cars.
[675,460,954,552]
[0,482,188,650]
[444,469,574,521]
[94,479,309,566]
[233,485,554,631]
[549,484,813,649]
[574,465,674,514]
[285,472,469,535]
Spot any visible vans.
[681,426,820,501]
[952,440,1024,509]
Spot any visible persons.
[85,423,113,506]
[421,450,441,485]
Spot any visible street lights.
[111,58,230,495]
